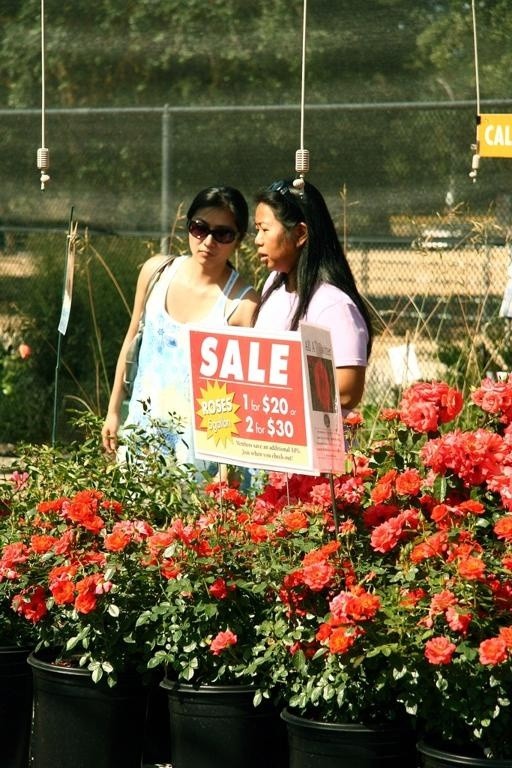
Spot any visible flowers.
[0,372,511,753]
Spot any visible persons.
[238,176,373,493]
[102,185,261,483]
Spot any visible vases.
[415,738,512,768]
[26,649,147,768]
[158,676,279,768]
[0,642,31,768]
[280,705,409,768]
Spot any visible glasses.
[265,180,299,208]
[189,221,242,244]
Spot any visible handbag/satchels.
[123,254,176,398]
